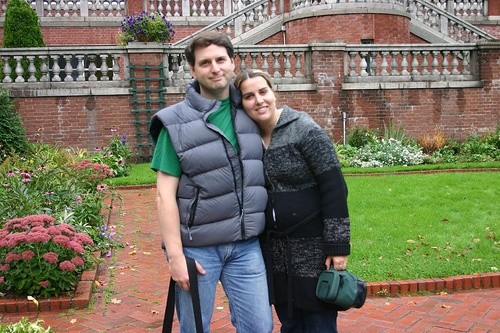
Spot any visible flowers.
[116,10,175,46]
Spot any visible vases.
[137,33,155,42]
